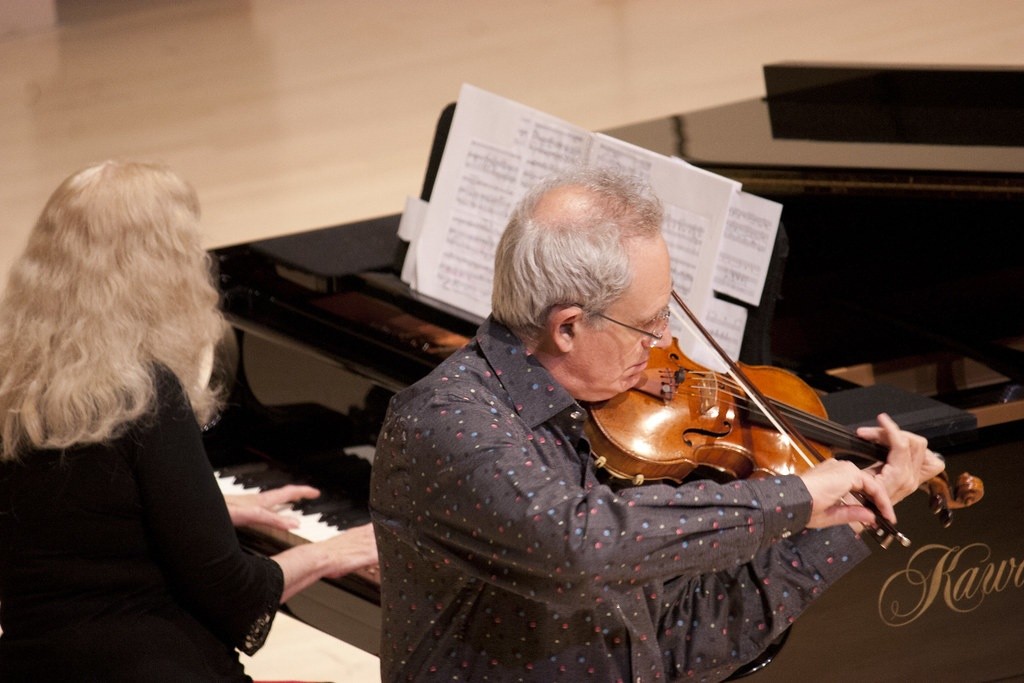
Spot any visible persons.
[372,176,945,683]
[0,161,379,683]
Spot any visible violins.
[583,334,985,530]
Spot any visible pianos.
[201,59,1024,683]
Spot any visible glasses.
[550,302,671,348]
